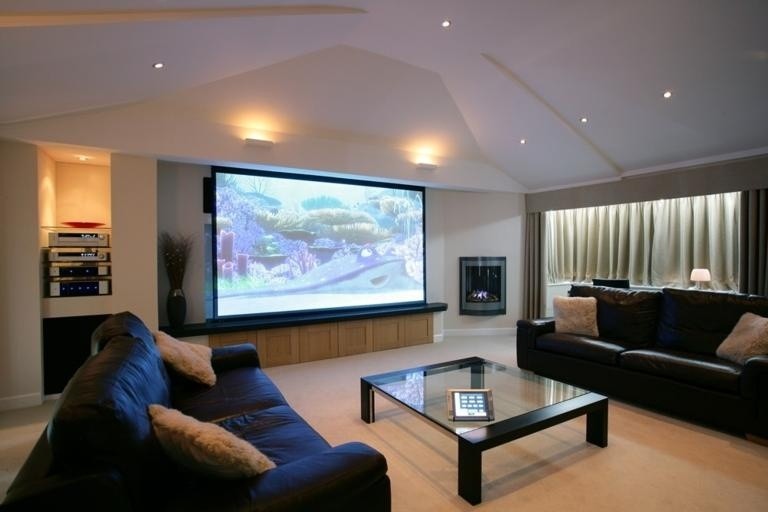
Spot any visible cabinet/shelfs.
[160,302,450,368]
[39,221,112,300]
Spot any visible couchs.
[1,311,393,512]
[512,280,768,444]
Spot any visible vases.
[164,287,189,325]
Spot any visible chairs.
[590,278,631,289]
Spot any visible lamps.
[690,267,712,290]
[415,161,437,171]
[243,136,275,148]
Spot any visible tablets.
[452,391,489,420]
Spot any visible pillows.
[150,324,218,390]
[713,310,768,365]
[145,401,271,479]
[550,292,604,341]
